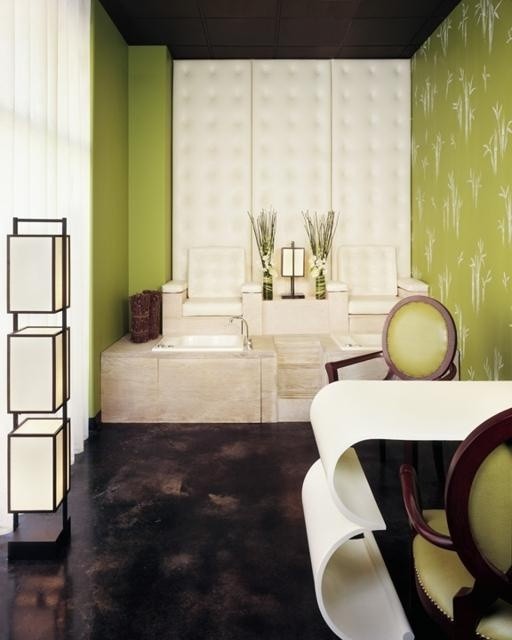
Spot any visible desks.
[263,299,329,336]
[301,380,512,614]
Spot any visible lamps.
[8,216,66,559]
[281,241,307,298]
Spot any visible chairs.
[327,242,429,336]
[161,235,262,335]
[325,295,458,384]
[398,408,512,639]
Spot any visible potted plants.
[301,210,340,300]
[244,206,280,299]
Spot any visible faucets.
[229,315,249,346]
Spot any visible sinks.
[151,331,249,353]
